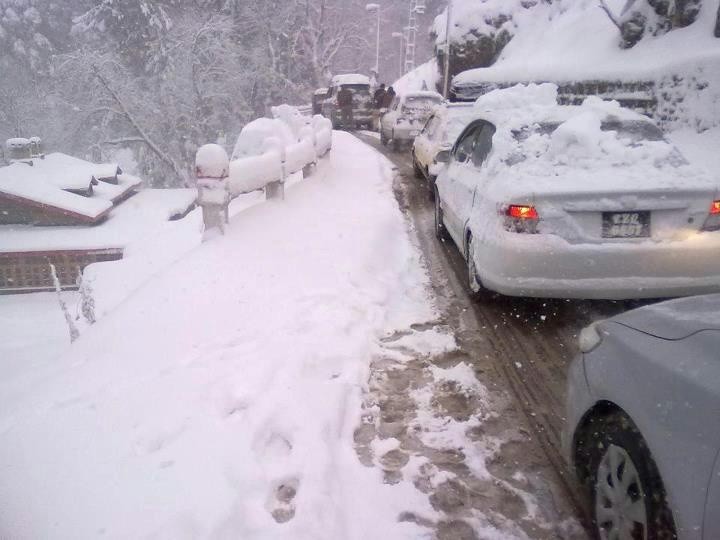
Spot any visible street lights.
[366,2,381,73]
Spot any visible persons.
[372,83,386,132]
[337,85,355,131]
[380,86,396,122]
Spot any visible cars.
[410,100,720,299]
[378,91,447,152]
[311,74,377,129]
[565,290,720,540]
